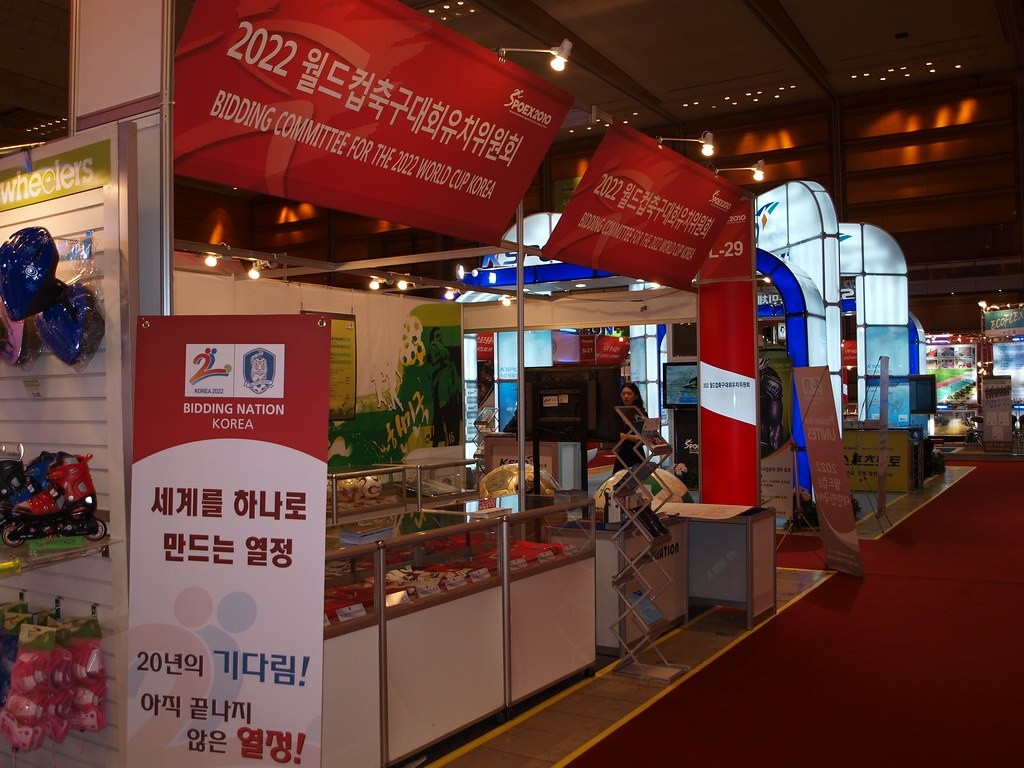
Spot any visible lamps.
[658,131,714,156]
[497,38,573,72]
[206,243,410,291]
[714,158,765,181]
[445,288,514,306]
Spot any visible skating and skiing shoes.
[2,452,108,546]
[0,450,59,534]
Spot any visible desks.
[486,431,778,660]
[842,428,923,494]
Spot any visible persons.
[612,382,649,479]
[758,356,784,455]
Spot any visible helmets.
[0,294,24,366]
[36,282,104,366]
[0,226,60,321]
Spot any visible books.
[630,504,671,545]
[612,463,641,492]
[626,587,664,624]
[631,416,673,453]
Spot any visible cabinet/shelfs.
[0,119,137,768]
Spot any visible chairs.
[966,414,1024,447]
